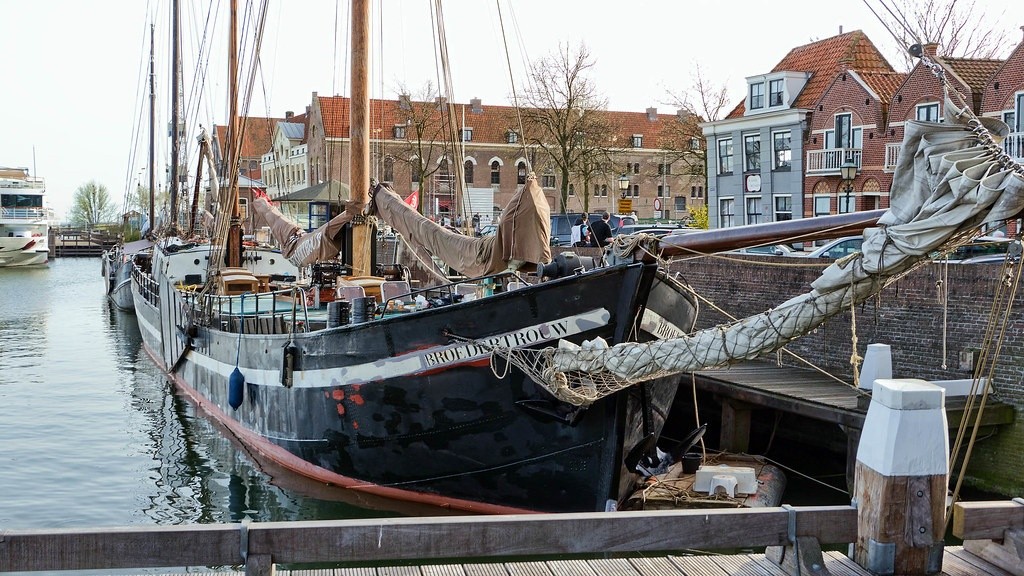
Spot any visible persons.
[585,211,613,247]
[570,213,589,247]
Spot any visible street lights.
[839,158,859,213]
[618,173,630,199]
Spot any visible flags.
[403,188,420,210]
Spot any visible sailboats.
[103,0,1024,516]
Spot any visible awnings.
[271,179,352,201]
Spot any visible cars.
[929,236,1024,263]
[612,225,700,237]
[807,235,864,258]
[731,242,810,257]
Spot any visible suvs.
[551,214,635,247]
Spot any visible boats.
[0,143,50,267]
